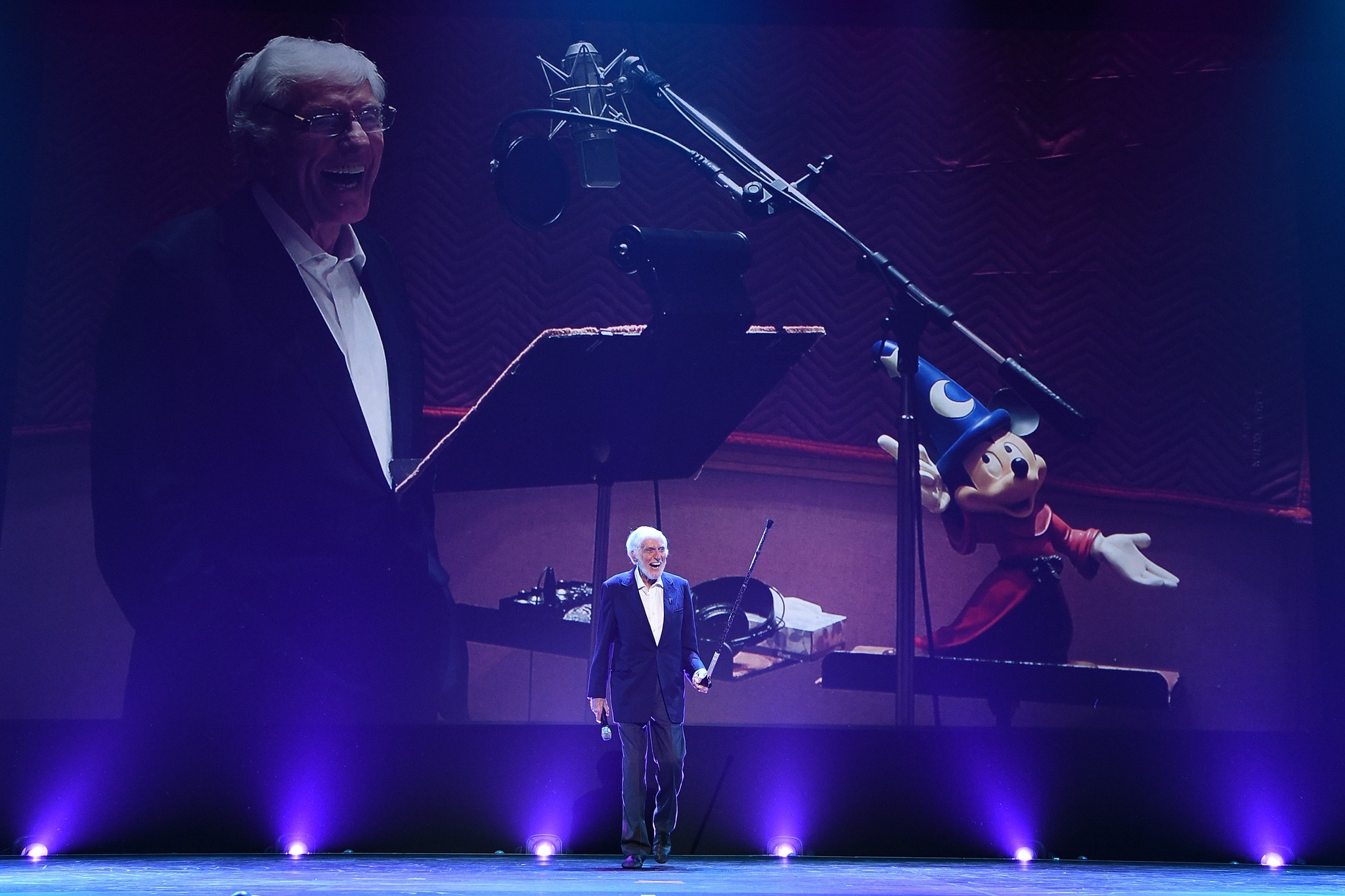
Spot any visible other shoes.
[652,831,672,863]
[621,854,644,869]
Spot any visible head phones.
[688,575,786,676]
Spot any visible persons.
[89,33,471,850]
[586,525,712,869]
[869,339,1182,663]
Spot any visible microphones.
[565,41,621,189]
[601,706,611,741]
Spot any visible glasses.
[256,99,398,137]
